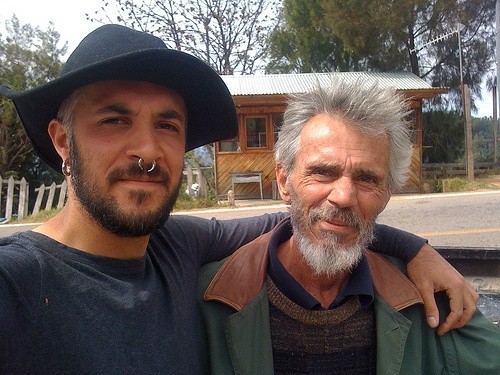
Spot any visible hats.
[0,23,239,176]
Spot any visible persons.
[192,72,500,375]
[1,23,483,374]
